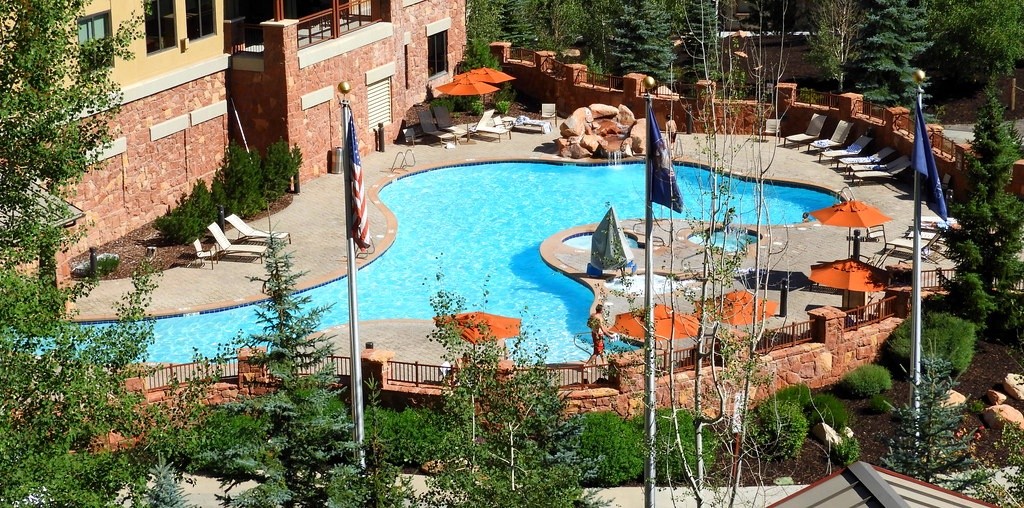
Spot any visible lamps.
[184,38,190,50]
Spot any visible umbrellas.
[451,66,516,111]
[692,291,778,328]
[432,312,521,363]
[435,73,502,142]
[808,258,894,309]
[811,196,894,257]
[608,304,699,350]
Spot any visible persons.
[665,114,677,144]
[583,305,615,372]
[453,352,472,368]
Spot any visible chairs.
[224,213,291,251]
[416,110,456,148]
[432,106,471,145]
[837,146,913,186]
[783,113,827,150]
[761,119,782,143]
[885,216,958,262]
[402,128,423,147]
[207,221,269,265]
[867,224,887,239]
[807,120,855,158]
[192,238,218,270]
[819,135,874,165]
[474,103,556,142]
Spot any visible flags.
[647,103,683,214]
[910,93,947,222]
[343,103,375,259]
